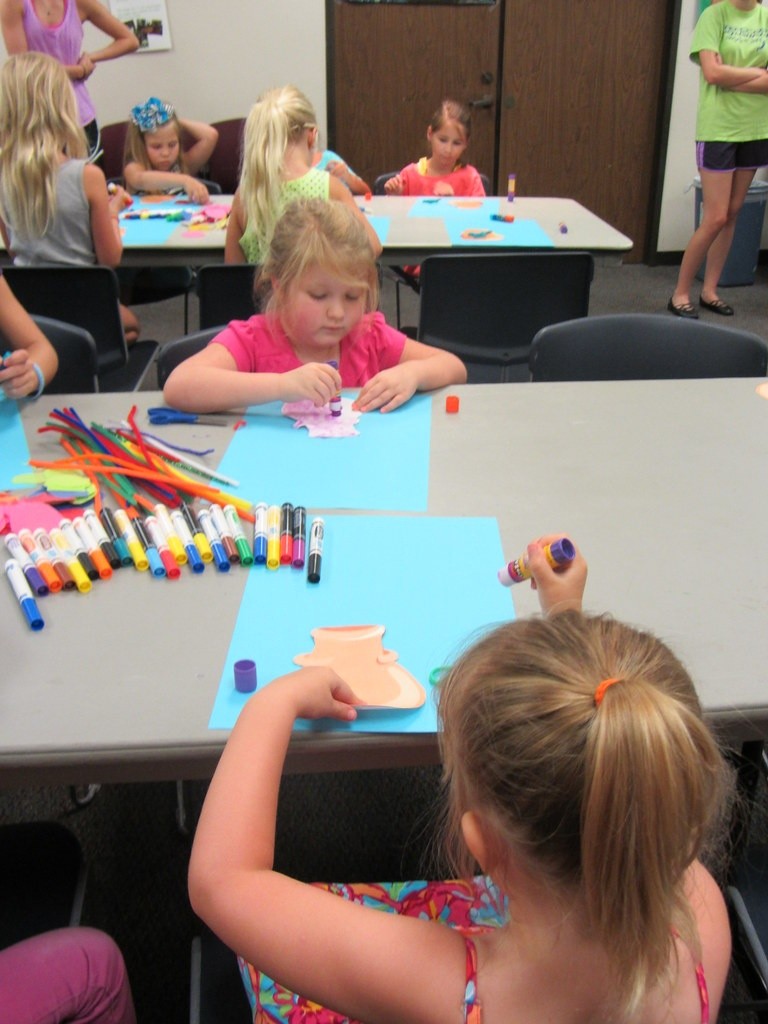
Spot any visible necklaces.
[37,0,57,15]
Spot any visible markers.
[180,501,214,564]
[292,507,306,569]
[308,517,324,583]
[112,509,149,572]
[253,502,268,565]
[222,505,254,567]
[4,517,113,631]
[153,503,188,565]
[143,516,181,580]
[280,503,293,565]
[266,506,281,569]
[107,182,194,222]
[196,509,231,572]
[82,509,121,570]
[170,511,205,574]
[98,507,133,568]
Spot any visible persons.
[225,86,382,265]
[123,97,219,204]
[384,99,486,276]
[0,53,140,347]
[0,926,136,1024]
[162,196,467,413]
[0,265,59,400]
[0,0,138,184]
[310,147,371,195]
[668,0,768,319]
[188,531,732,1024]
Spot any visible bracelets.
[26,362,45,401]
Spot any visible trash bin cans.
[691,176,768,287]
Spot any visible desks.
[0,374,768,835]
[106,192,634,267]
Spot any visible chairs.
[1,119,767,1024]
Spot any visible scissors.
[148,407,227,427]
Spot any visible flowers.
[130,97,174,131]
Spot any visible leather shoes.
[699,295,734,315]
[667,296,699,319]
